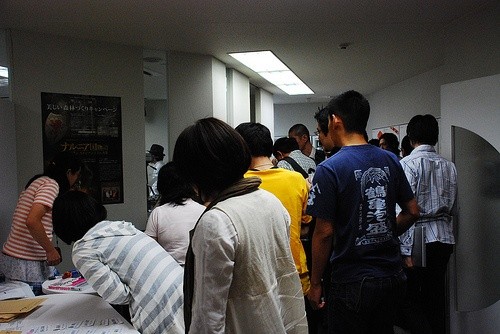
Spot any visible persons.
[272,105,341,185]
[364,131,415,161]
[0,156,82,292]
[235,121,311,303]
[308,91,420,334]
[146,143,165,211]
[396,114,457,334]
[171,116,308,334]
[51,190,185,334]
[144,162,207,269]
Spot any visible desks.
[0,294,140,334]
[0,280,35,301]
[41,275,97,295]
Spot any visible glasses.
[314,130,323,135]
